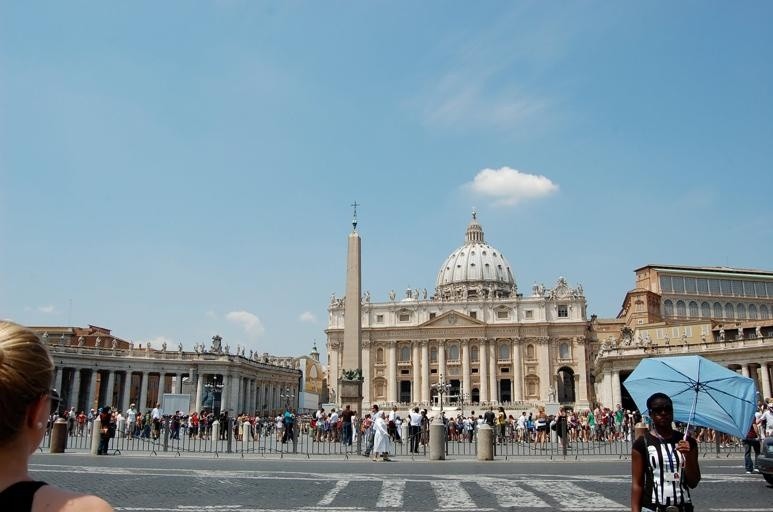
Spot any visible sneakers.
[362,453,391,462]
[746,469,760,474]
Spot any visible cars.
[755,431,772,485]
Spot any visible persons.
[330,277,584,299]
[600,324,763,349]
[630,392,702,511]
[47,398,773,474]
[0,322,115,511]
[42,330,299,369]
[673,473,680,479]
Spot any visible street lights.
[279,387,295,408]
[430,373,452,412]
[203,375,224,417]
[454,386,470,418]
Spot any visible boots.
[97,448,108,455]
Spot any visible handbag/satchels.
[100,429,108,433]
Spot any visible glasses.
[649,406,673,414]
[39,388,62,414]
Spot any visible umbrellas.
[620,354,760,442]
